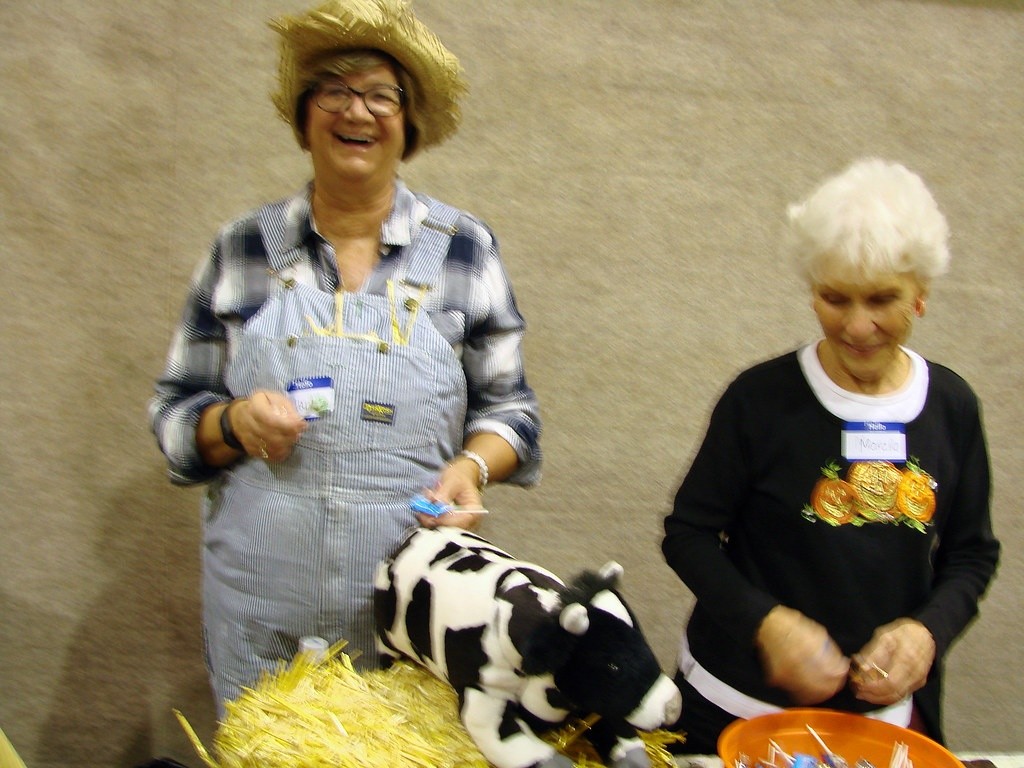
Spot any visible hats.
[265,1,468,162]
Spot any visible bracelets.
[221,400,246,452]
[461,449,488,485]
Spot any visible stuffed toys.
[372,524,682,768]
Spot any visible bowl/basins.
[717,709,966,768]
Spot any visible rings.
[259,440,270,458]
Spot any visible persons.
[660,159,1001,758]
[149,0,542,718]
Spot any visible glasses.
[312,79,405,118]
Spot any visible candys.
[737,722,879,768]
[409,496,453,517]
